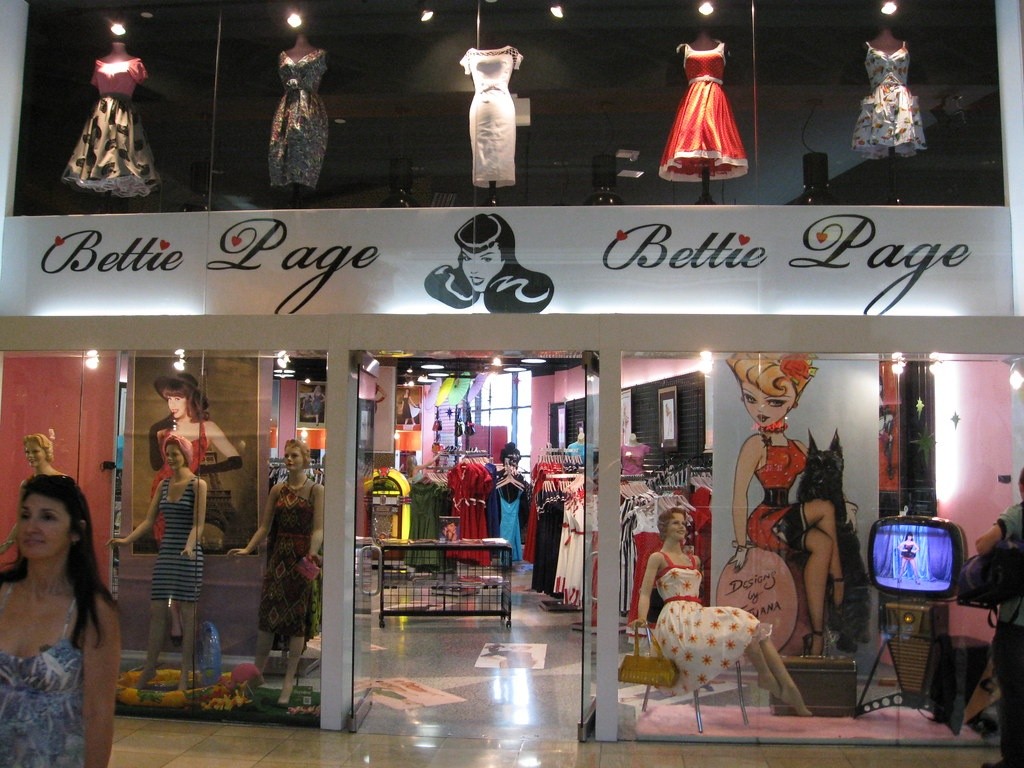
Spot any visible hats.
[154,373,209,410]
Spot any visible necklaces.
[287,477,308,494]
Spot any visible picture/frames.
[658,386,678,452]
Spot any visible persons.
[627,505,812,717]
[895,533,921,586]
[0,472,122,768]
[61,32,931,201]
[104,433,209,696]
[564,432,585,466]
[375,383,387,414]
[974,466,1024,768]
[371,644,538,710]
[169,597,184,647]
[311,385,325,426]
[620,432,652,476]
[148,372,244,554]
[399,388,419,426]
[227,438,324,707]
[0,432,66,555]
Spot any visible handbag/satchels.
[957,502,1024,628]
[299,553,321,580]
[618,618,677,688]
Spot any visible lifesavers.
[115,664,238,709]
[196,623,225,687]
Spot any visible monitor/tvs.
[868,515,968,598]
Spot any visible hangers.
[460,451,489,467]
[535,447,583,467]
[415,465,448,486]
[540,473,584,494]
[619,465,712,517]
[496,464,524,491]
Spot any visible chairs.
[641,622,749,733]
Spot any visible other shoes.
[916,579,920,585]
[898,578,902,583]
[277,699,289,706]
[981,760,1004,768]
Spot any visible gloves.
[194,456,243,476]
[149,412,178,471]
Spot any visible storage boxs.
[769,652,858,718]
